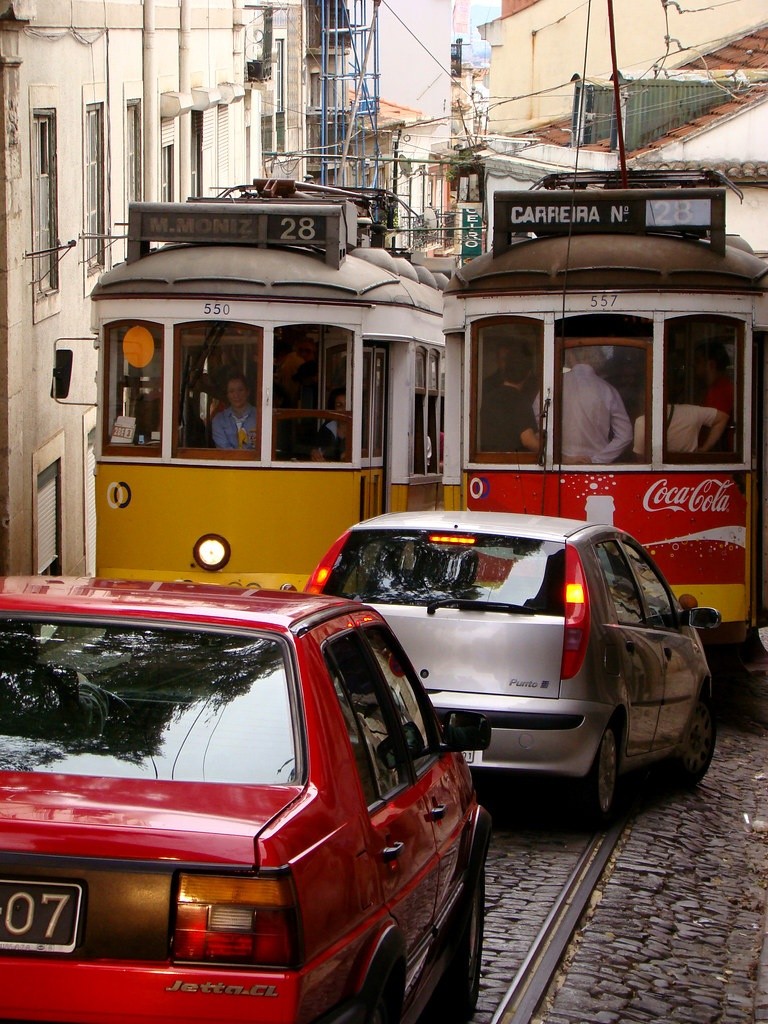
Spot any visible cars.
[0,573,494,1024]
[300,509,724,829]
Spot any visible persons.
[135,395,159,440]
[689,340,734,450]
[633,376,728,464]
[200,343,258,449]
[211,372,258,450]
[480,347,593,465]
[310,395,351,462]
[284,335,319,456]
[532,343,635,463]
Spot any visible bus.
[44,173,445,591]
[444,163,768,661]
